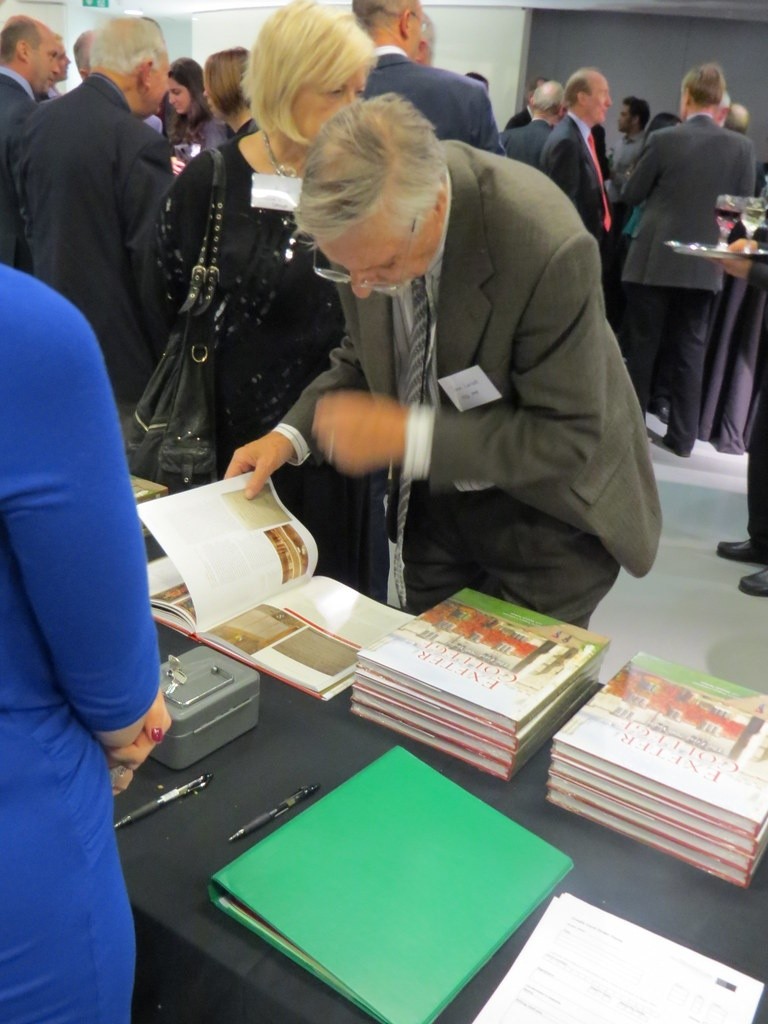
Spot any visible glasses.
[312,217,418,299]
[411,9,430,32]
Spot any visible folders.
[207,746,575,1024]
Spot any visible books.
[137,472,767,1024]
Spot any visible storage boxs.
[148,646,262,771]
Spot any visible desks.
[111,619,768,1024]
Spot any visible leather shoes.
[717,538,768,564]
[739,569,768,598]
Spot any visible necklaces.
[262,130,298,177]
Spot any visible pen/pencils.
[113,773,214,829]
[229,784,321,842]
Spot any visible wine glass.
[714,194,742,252]
[740,197,766,239]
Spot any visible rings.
[326,433,336,464]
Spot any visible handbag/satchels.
[123,147,227,496]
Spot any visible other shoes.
[663,435,690,457]
[657,406,669,425]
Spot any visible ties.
[392,277,430,610]
[588,133,611,232]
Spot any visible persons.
[1,12,252,442]
[353,0,768,597]
[225,92,663,630]
[0,262,171,1024]
[145,2,387,603]
